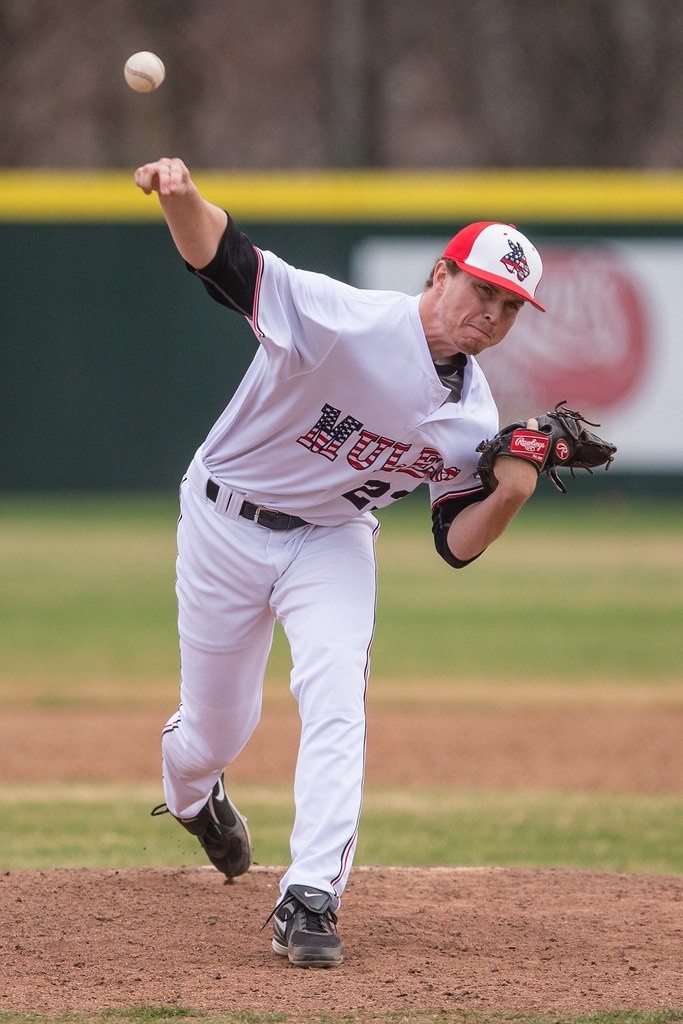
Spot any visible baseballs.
[125,51,166,93]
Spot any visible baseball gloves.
[477,410,617,496]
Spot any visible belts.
[205,479,308,531]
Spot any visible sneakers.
[261,885,343,966]
[151,773,252,876]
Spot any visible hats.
[441,222,546,312]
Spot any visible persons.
[130,156,617,965]
